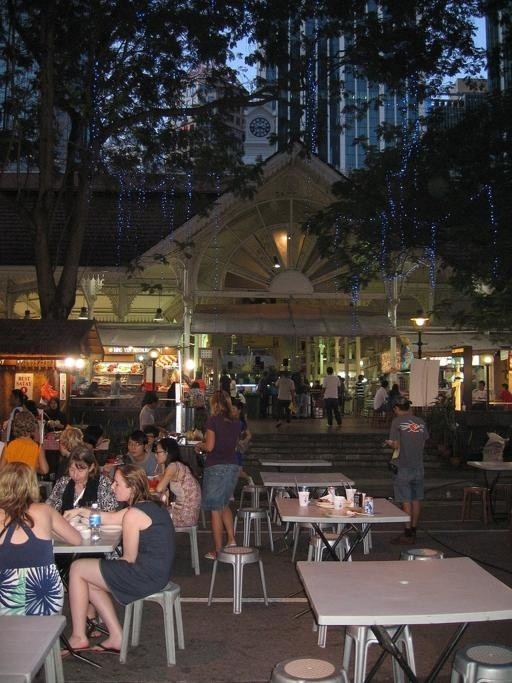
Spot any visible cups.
[298,491,310,506]
[148,476,157,492]
[70,521,92,539]
[317,487,374,513]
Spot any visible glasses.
[153,450,167,456]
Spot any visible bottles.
[90,503,103,543]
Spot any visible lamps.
[20,291,166,321]
[273,256,281,269]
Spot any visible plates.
[100,524,122,532]
[326,510,355,517]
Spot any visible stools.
[363,393,393,426]
[120,581,186,667]
[173,524,200,576]
[206,544,269,616]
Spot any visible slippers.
[93,641,122,656]
[89,618,102,640]
[205,551,217,559]
[59,642,90,659]
[225,541,237,549]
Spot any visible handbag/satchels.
[237,439,253,453]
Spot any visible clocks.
[248,116,271,139]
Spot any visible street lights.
[148,346,161,396]
[483,354,495,407]
[407,310,433,359]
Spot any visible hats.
[391,396,412,405]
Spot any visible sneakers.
[336,424,342,426]
[391,533,417,545]
[286,422,292,426]
[274,420,282,427]
[325,424,332,427]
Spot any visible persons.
[253,366,451,430]
[3,368,253,656]
[492,383,512,410]
[471,380,487,410]
[383,397,432,545]
